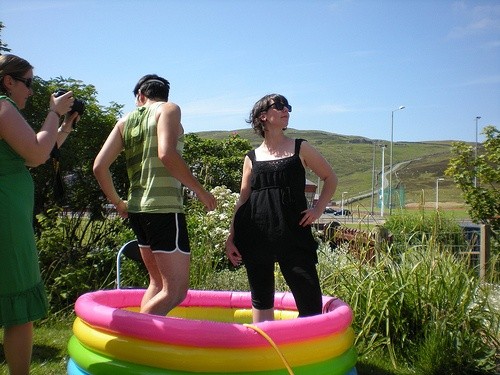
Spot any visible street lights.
[389,106,405,215]
[436,178,445,213]
[475,116,481,188]
[341,191,348,216]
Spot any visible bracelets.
[59,127,71,134]
[47,108,61,117]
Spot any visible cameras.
[53,88,86,117]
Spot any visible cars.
[326,208,350,216]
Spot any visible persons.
[92,74,218,316]
[0,54,80,375]
[225,93,338,324]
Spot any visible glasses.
[10,75,34,90]
[262,102,292,113]
